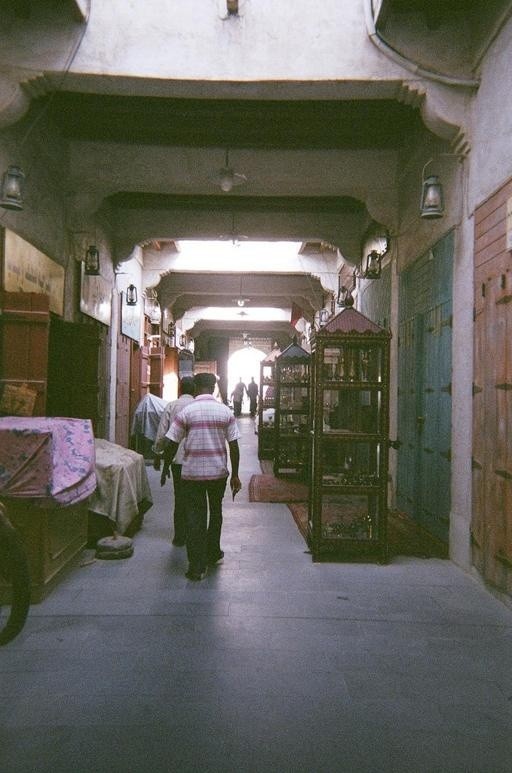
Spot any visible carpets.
[250,472,436,559]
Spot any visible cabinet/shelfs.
[258,288,394,566]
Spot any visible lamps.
[126,283,143,309]
[216,206,250,246]
[419,157,449,220]
[229,274,252,309]
[364,245,382,280]
[203,149,252,195]
[84,244,104,277]
[1,160,27,212]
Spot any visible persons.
[152,375,196,546]
[160,372,242,580]
[230,386,244,417]
[247,377,258,415]
[237,377,246,399]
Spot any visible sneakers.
[185,570,204,582]
[173,539,184,546]
[211,551,224,566]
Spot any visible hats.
[193,372,215,386]
